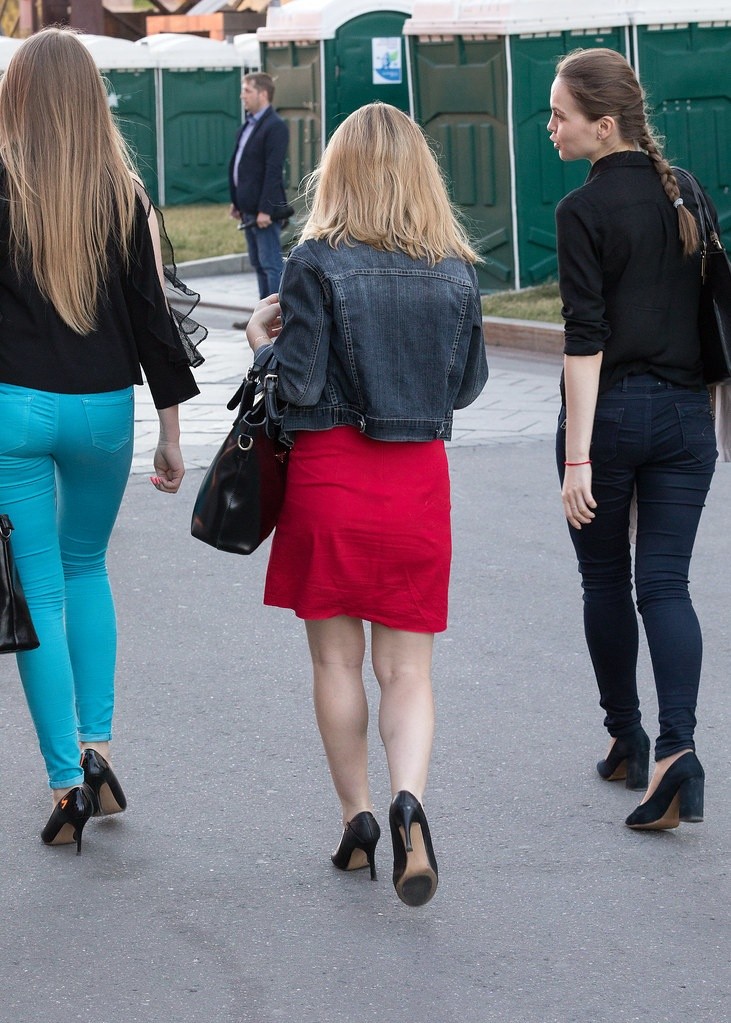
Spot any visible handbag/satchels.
[0,513,39,654]
[190,345,289,555]
[668,166,731,384]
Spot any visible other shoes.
[233,318,251,330]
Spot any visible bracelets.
[254,335,272,351]
[565,458,590,469]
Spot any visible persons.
[246,102,488,906]
[545,47,731,830]
[0,27,208,855]
[227,72,294,329]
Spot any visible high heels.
[626,753,705,830]
[596,726,650,791]
[388,790,437,908]
[331,811,380,882]
[79,748,126,816]
[41,786,92,855]
